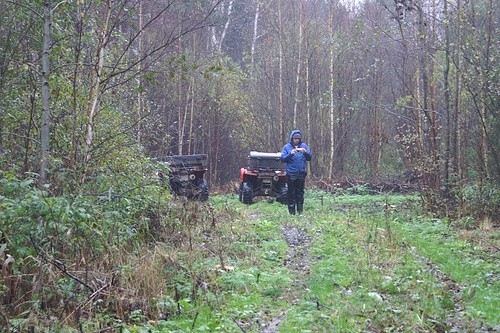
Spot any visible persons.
[280,130,312,216]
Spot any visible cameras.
[295,148,303,153]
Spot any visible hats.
[292,133,302,139]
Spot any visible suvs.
[238,151,289,204]
[161,153,210,202]
